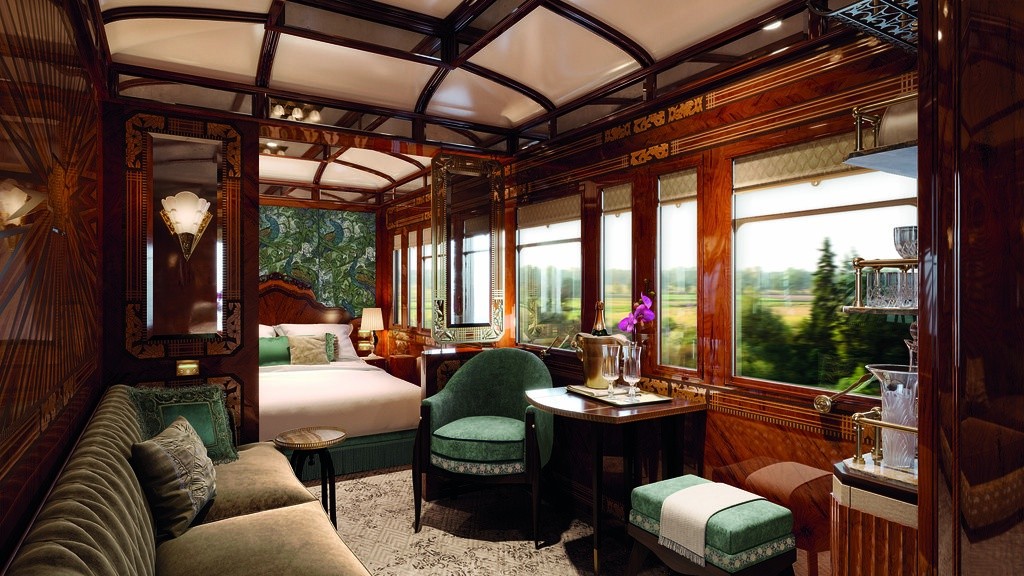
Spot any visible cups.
[894,225,918,259]
[866,272,918,309]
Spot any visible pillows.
[258,324,276,339]
[125,383,239,466]
[132,415,217,538]
[273,324,366,364]
[287,335,330,365]
[258,333,335,366]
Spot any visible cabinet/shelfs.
[807,0,919,316]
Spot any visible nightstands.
[359,356,385,371]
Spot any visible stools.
[713,456,832,576]
[274,425,346,532]
[629,473,798,576]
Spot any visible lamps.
[361,308,385,358]
[161,191,211,261]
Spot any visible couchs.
[0,374,373,576]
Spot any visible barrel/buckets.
[577,334,619,389]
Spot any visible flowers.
[616,291,657,345]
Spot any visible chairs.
[412,347,553,551]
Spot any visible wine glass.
[623,346,642,400]
[602,344,620,400]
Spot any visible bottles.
[591,301,608,336]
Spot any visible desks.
[524,387,707,576]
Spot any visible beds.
[258,272,422,482]
